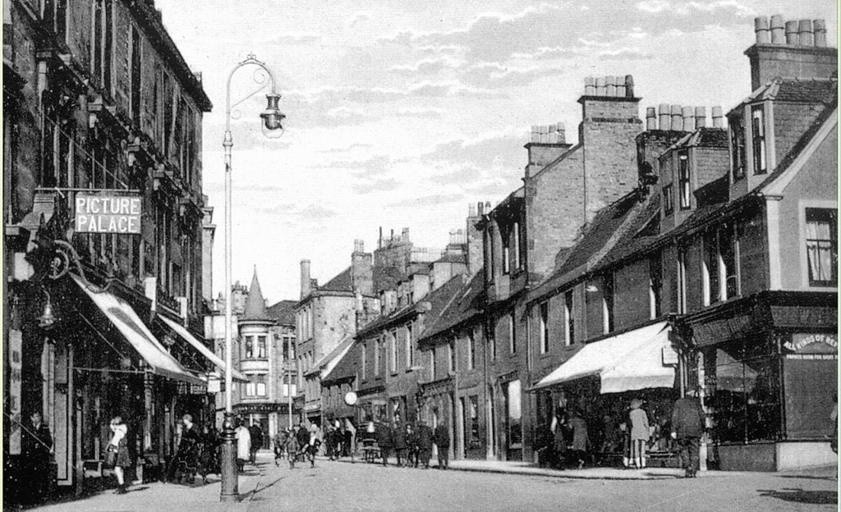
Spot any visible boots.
[642,458,645,469]
[635,457,640,469]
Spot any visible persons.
[170,409,456,486]
[105,414,135,495]
[533,390,705,477]
[25,410,54,508]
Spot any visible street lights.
[217,51,291,503]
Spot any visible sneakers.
[289,466,293,469]
[335,457,339,460]
[311,463,314,467]
[329,457,333,460]
[383,461,448,469]
[117,485,126,494]
[692,470,698,477]
[685,469,692,477]
